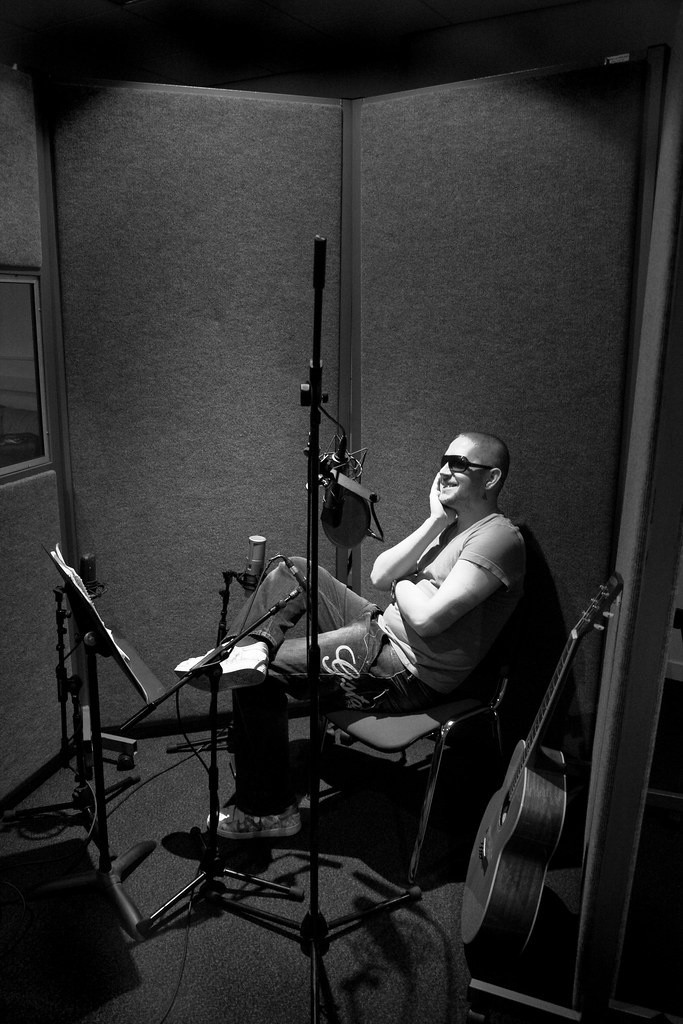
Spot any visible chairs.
[327,527,532,891]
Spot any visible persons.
[174,431,525,837]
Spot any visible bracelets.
[390,577,413,602]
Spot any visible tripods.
[0,233,422,1024]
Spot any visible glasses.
[440,455,492,473]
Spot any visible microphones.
[243,535,267,597]
[325,435,348,480]
[283,557,312,594]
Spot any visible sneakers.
[206,805,301,839]
[174,636,268,691]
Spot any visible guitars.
[460,572,627,951]
